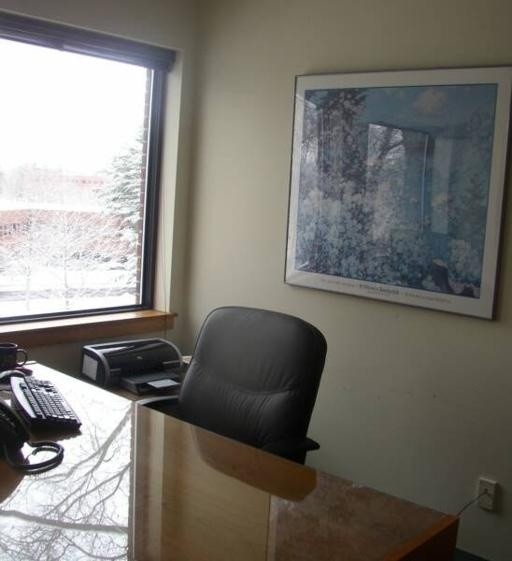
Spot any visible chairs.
[134,304,328,465]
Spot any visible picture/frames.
[283,62,512,323]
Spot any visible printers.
[81,337,183,395]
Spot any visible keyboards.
[9,375,83,429]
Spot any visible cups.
[0,341,28,371]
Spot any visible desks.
[0,356,462,561]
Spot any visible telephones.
[0,396,31,455]
